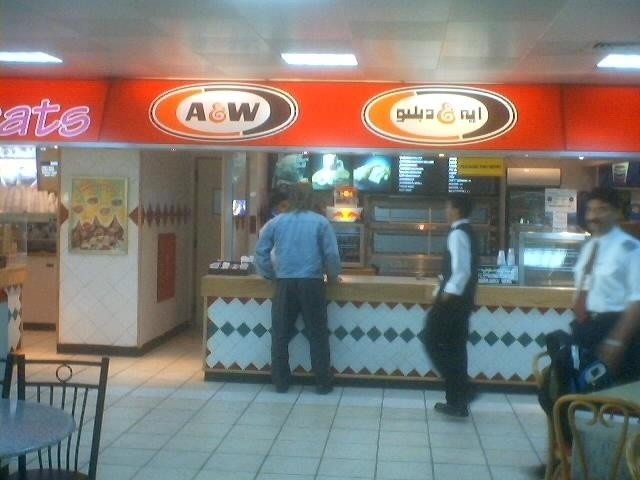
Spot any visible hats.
[582,186,624,210]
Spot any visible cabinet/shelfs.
[331,168,508,278]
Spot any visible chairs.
[0,352,110,480]
[531,350,639,480]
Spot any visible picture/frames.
[68,177,129,256]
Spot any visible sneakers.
[432,402,470,418]
[274,382,334,395]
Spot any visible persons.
[536,184,639,445]
[417,186,479,422]
[267,193,294,219]
[256,175,344,397]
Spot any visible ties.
[573,241,601,326]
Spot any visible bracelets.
[602,338,631,351]
